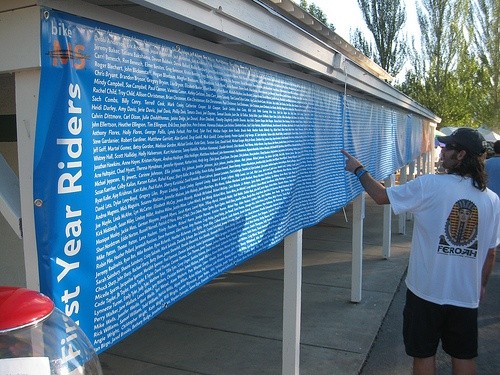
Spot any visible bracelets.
[353,165,369,181]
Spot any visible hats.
[436,128,487,156]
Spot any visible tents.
[435,126,500,149]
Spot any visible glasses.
[445,144,456,150]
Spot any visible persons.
[484,140,500,197]
[339,128,499,375]
